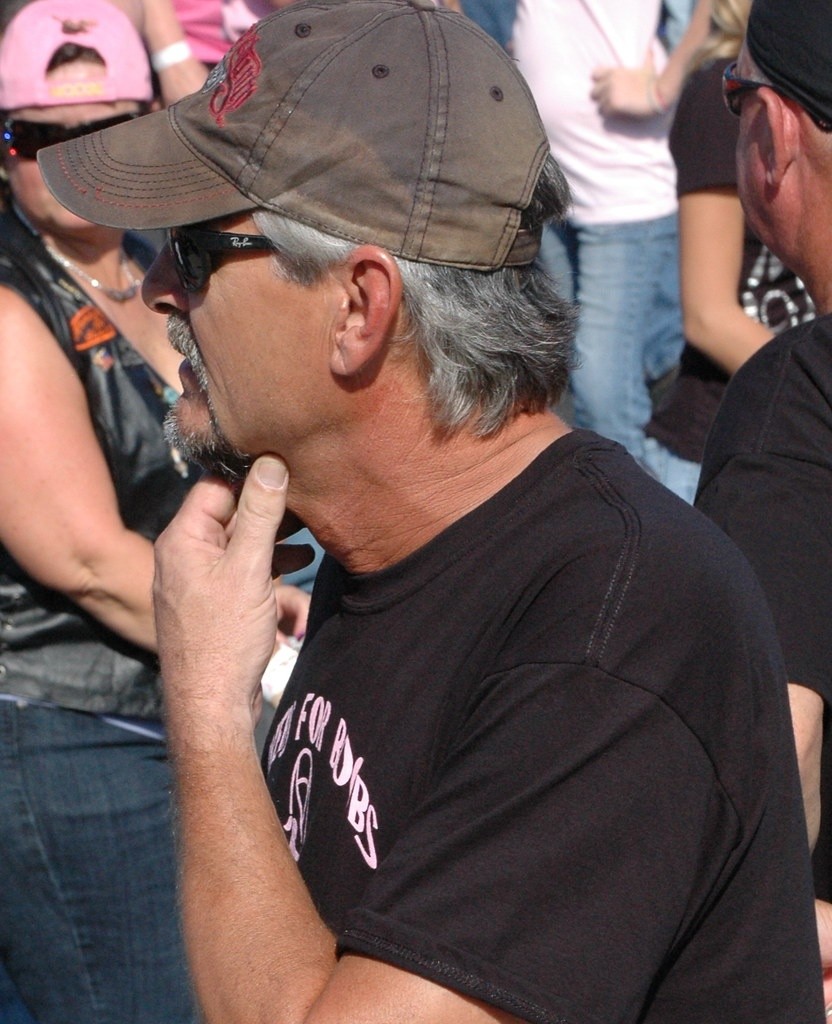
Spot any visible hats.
[747,0,832,124]
[36,0,553,271]
[0,0,153,110]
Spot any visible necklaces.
[46,244,136,302]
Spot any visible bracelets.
[261,645,298,702]
[649,80,670,116]
[151,40,191,73]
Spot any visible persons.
[0,0,832,1024]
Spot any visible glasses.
[162,227,277,293]
[0,111,151,164]
[721,61,832,132]
